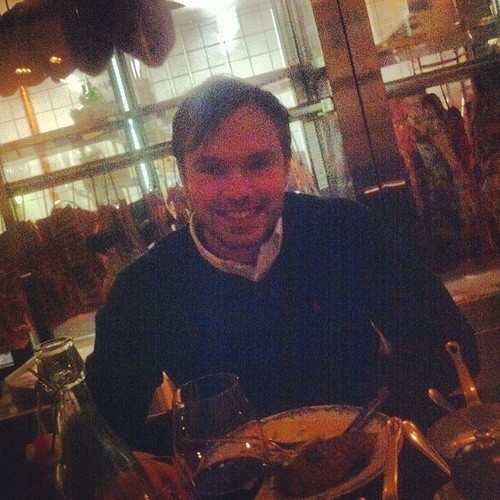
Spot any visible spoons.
[182,434,309,455]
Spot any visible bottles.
[34,335,160,500]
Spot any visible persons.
[398,89,480,262]
[24,73,479,500]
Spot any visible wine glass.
[171,373,265,500]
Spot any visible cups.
[101,457,195,500]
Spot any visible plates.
[197,405,403,500]
